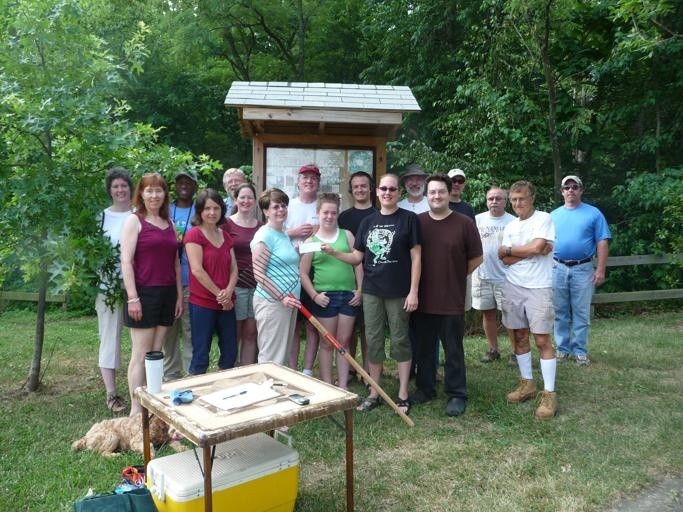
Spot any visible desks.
[133,359,365,512]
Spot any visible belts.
[553,257,590,266]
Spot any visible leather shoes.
[446,398,465,416]
[395,389,436,416]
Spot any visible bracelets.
[505,244,512,255]
[126,297,141,304]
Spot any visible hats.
[402,165,427,177]
[175,170,197,182]
[299,165,321,176]
[561,175,582,186]
[447,169,465,179]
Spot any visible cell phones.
[288,393,310,405]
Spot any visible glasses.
[380,186,397,191]
[563,185,578,189]
[452,178,464,184]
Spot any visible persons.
[120,173,184,417]
[162,171,198,383]
[549,175,612,365]
[250,187,301,434]
[95,167,137,412]
[219,183,265,368]
[498,179,558,421]
[472,186,519,367]
[221,168,262,222]
[184,187,238,378]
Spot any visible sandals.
[335,371,381,413]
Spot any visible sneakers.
[480,350,500,362]
[536,391,557,419]
[506,378,536,403]
[107,395,126,411]
[556,353,590,366]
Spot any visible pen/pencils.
[223,390,247,399]
[273,384,288,386]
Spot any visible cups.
[144,351,164,394]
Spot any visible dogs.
[70,410,188,461]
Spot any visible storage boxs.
[143,433,299,511]
